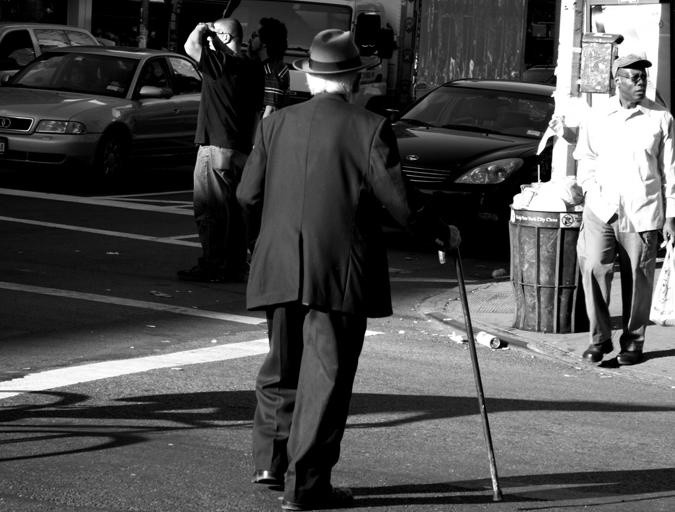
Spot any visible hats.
[611,54,651,78]
[291,29,374,75]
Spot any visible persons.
[566,55,675,366]
[249,17,291,117]
[174,16,259,284]
[235,28,466,510]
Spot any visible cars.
[382,76,557,245]
[0,45,209,190]
[520,65,557,86]
[0,21,103,90]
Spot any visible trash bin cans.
[508,204,590,334]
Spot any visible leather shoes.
[583,338,613,363]
[251,470,354,510]
[616,350,643,365]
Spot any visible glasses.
[632,74,646,81]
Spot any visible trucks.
[215,1,526,115]
